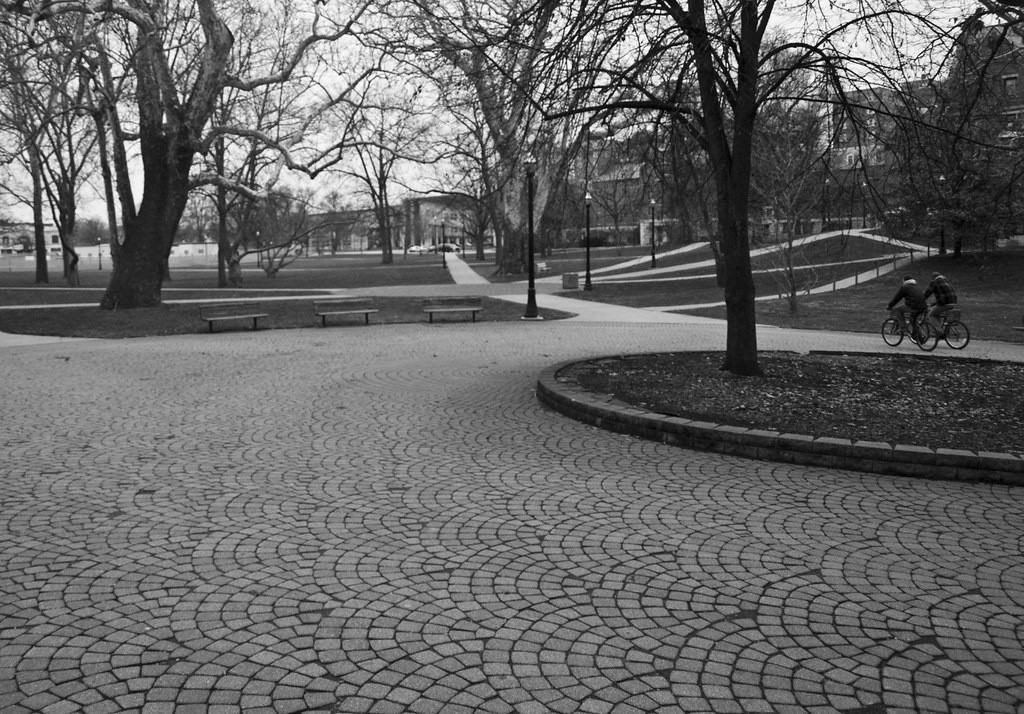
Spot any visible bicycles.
[880,306,939,352]
[906,303,971,350]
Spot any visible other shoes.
[919,334,927,344]
[896,328,907,332]
[937,334,946,339]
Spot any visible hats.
[931,271,940,279]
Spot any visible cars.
[409,243,461,253]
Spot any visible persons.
[922,271,958,340]
[888,276,927,335]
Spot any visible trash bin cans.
[562,273,578,289]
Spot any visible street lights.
[440,219,447,270]
[939,175,946,255]
[824,178,831,232]
[861,182,869,229]
[583,191,592,291]
[255,230,260,268]
[521,154,545,321]
[649,198,658,270]
[97,237,103,271]
[434,216,439,254]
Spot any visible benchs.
[199,301,268,333]
[312,298,379,326]
[421,296,483,324]
